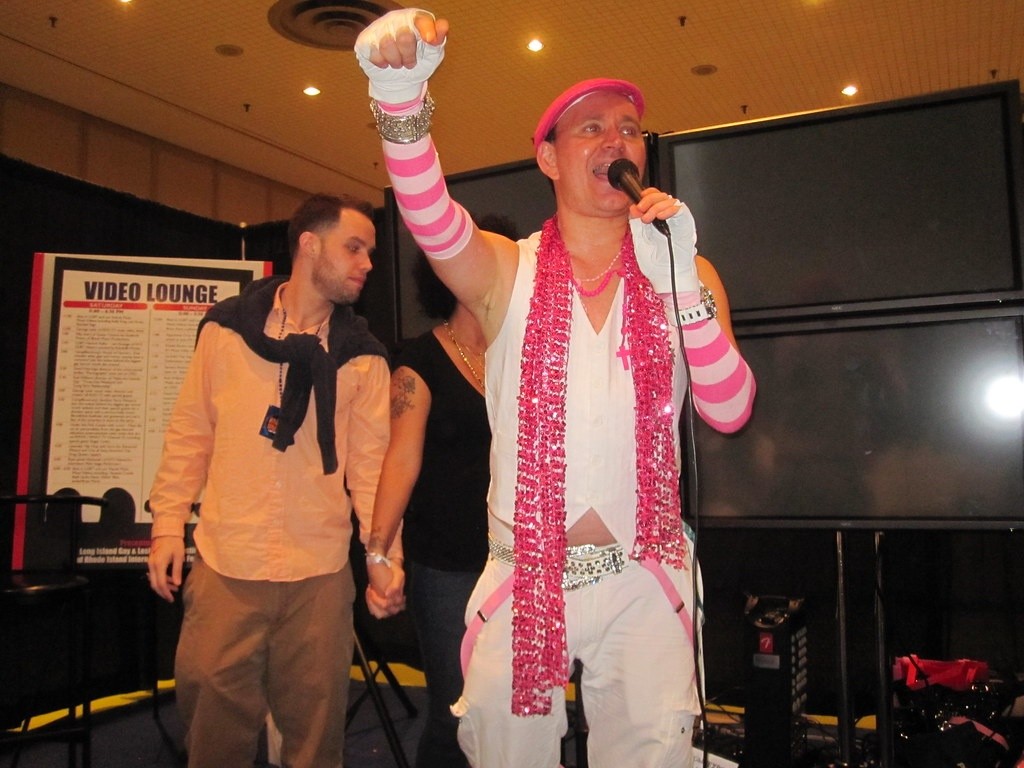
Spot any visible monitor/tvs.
[384,78,1024,531]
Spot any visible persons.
[144,190,410,767]
[361,213,523,768]
[354,7,760,768]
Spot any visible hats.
[532,76,647,144]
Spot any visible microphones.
[608,153,678,238]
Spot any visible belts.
[487,531,635,592]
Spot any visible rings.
[146,572,150,582]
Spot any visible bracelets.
[668,279,717,326]
[369,92,434,143]
[367,552,391,567]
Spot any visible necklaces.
[444,319,486,391]
[280,302,322,407]
[572,240,626,296]
